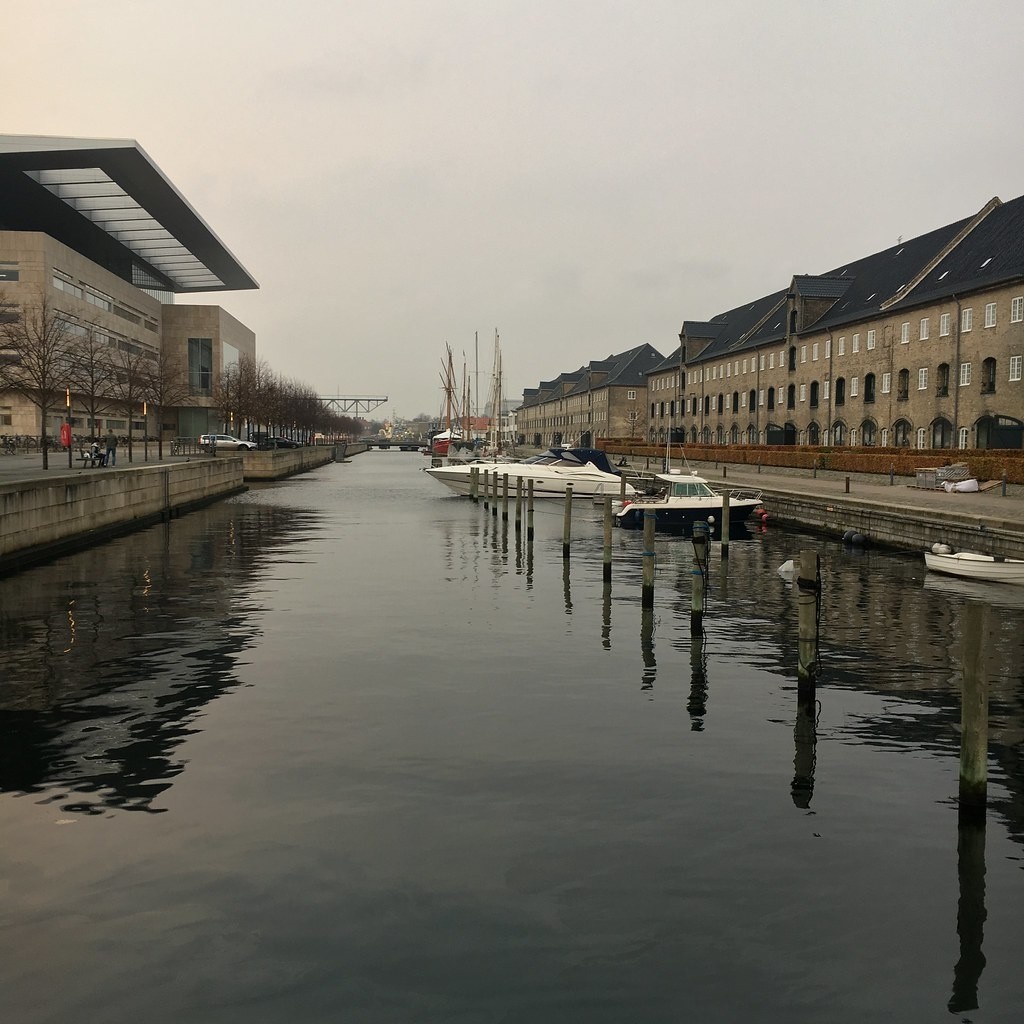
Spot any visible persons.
[91,438,105,467]
[103,429,117,468]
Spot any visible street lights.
[142,400,148,462]
[66,385,73,468]
[231,412,234,436]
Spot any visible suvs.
[198,434,258,453]
[263,436,300,449]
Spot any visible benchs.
[76,458,102,468]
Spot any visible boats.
[922,550,1024,587]
[609,414,764,526]
[420,421,656,500]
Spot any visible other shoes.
[104,463,106,467]
[111,465,116,468]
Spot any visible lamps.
[692,471,697,477]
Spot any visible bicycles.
[0,435,129,456]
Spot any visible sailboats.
[416,328,525,466]
